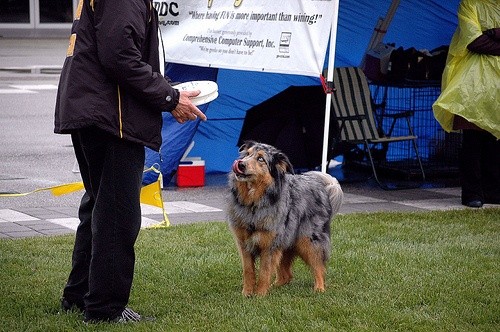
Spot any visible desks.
[366,74,450,162]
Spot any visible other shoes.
[466,198,483,207]
[485,196,500,204]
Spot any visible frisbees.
[171,81,219,106]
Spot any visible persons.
[54,0,207,325]
[433,0,500,208]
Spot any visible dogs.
[225,140,343,300]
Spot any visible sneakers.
[58,295,85,316]
[82,304,154,327]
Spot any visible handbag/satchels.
[389,45,451,87]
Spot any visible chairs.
[318,66,433,190]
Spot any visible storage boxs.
[177,141,206,187]
